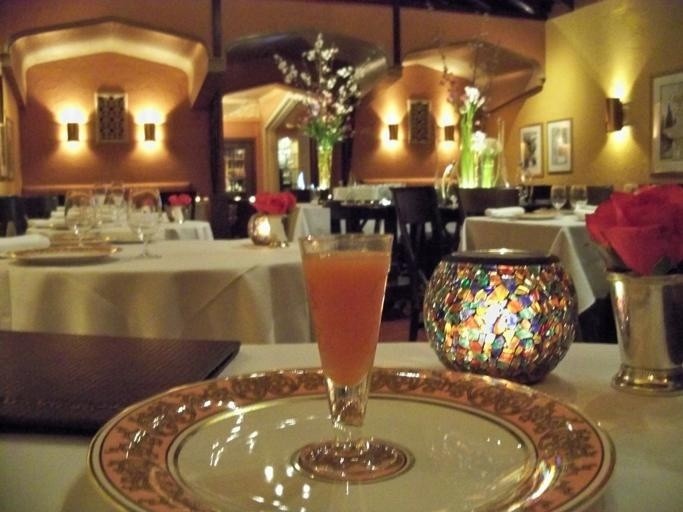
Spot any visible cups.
[600,265,683,395]
[291,230,412,483]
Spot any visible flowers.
[273,32,382,146]
[470,130,500,161]
[434,13,499,125]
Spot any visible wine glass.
[62,183,186,261]
[549,180,589,221]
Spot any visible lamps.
[145,123,155,140]
[389,125,398,139]
[67,123,79,140]
[605,98,623,132]
[445,126,454,141]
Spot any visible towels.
[0,331,240,435]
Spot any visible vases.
[606,273,683,398]
[458,128,479,188]
[479,161,497,188]
[316,145,332,190]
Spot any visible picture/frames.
[650,70,683,176]
[546,119,573,174]
[518,123,544,178]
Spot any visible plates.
[519,213,554,220]
[12,241,123,264]
[88,364,620,510]
